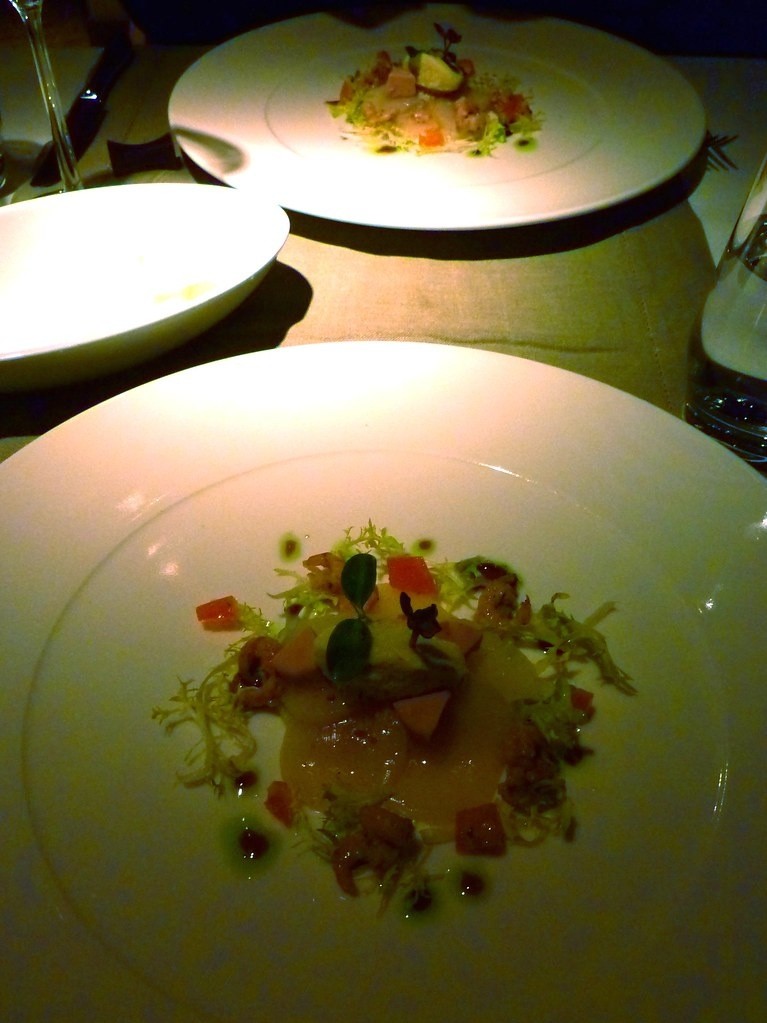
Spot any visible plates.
[0,341,767,1023]
[1,182,291,387]
[168,6,705,230]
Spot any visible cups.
[700,155,767,384]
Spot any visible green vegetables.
[327,22,542,156]
[149,520,639,919]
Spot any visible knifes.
[24,40,126,183]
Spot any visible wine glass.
[2,0,88,193]
